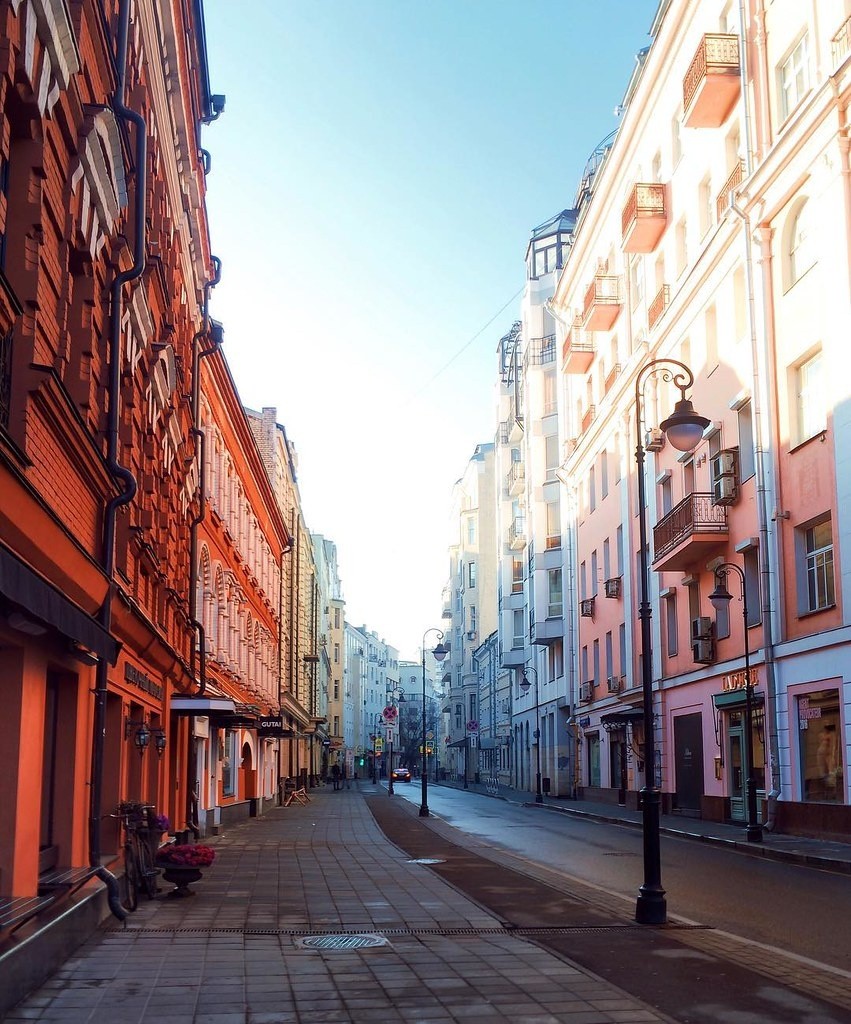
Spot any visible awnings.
[599,705,644,722]
[170,694,236,715]
[447,738,468,747]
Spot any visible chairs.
[39,843,103,895]
[0,895,54,935]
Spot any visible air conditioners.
[692,617,711,640]
[713,453,734,481]
[581,601,591,616]
[693,640,712,662]
[714,477,736,506]
[645,429,661,452]
[607,675,619,693]
[578,684,590,702]
[606,581,617,597]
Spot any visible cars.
[392,768,411,782]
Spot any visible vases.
[155,862,206,898]
[140,827,168,873]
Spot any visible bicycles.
[94,805,162,910]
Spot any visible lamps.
[124,715,152,763]
[150,725,167,761]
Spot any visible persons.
[817,719,840,799]
[332,761,341,791]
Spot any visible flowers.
[154,813,170,829]
[155,843,216,869]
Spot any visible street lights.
[520,667,544,804]
[633,360,713,922]
[372,712,383,784]
[447,701,468,790]
[427,721,439,784]
[419,627,449,816]
[707,563,764,845]
[388,686,407,796]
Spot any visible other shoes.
[334,788,337,791]
[337,788,340,790]
